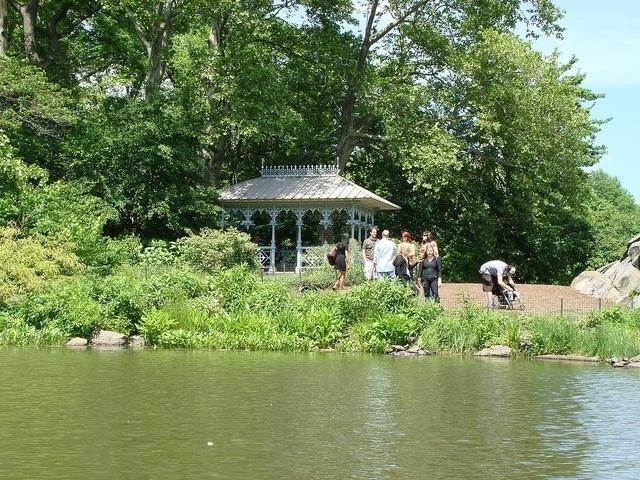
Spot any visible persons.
[420,229,439,258]
[478,259,516,309]
[373,228,397,282]
[362,226,380,282]
[392,242,425,297]
[417,244,443,305]
[332,233,350,291]
[397,231,416,263]
[499,277,520,304]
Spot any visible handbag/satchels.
[327,242,338,266]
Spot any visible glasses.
[422,235,428,236]
[402,236,406,238]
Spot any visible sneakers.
[333,285,347,291]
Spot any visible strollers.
[492,275,526,312]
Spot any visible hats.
[507,266,516,276]
